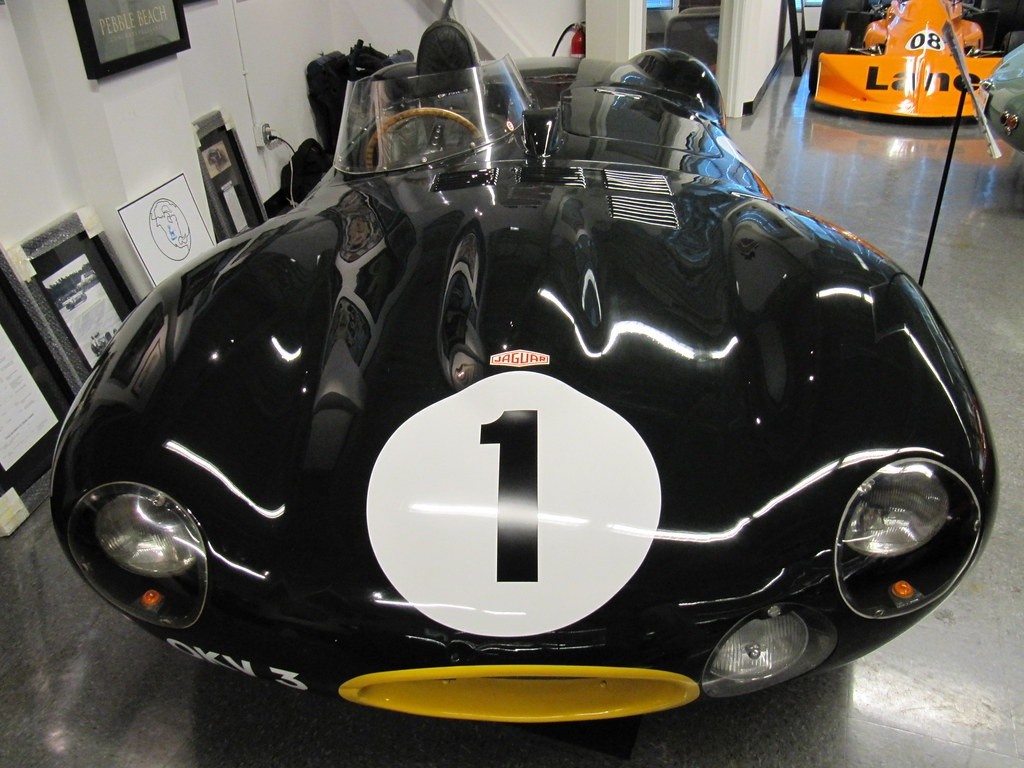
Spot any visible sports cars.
[47,1,999,723]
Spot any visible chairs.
[386,18,519,186]
[550,47,724,174]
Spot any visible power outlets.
[262,122,272,143]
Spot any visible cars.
[981,41,1023,154]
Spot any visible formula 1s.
[807,1,1004,125]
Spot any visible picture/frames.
[68,0,191,80]
[193,105,269,243]
[0,202,150,405]
